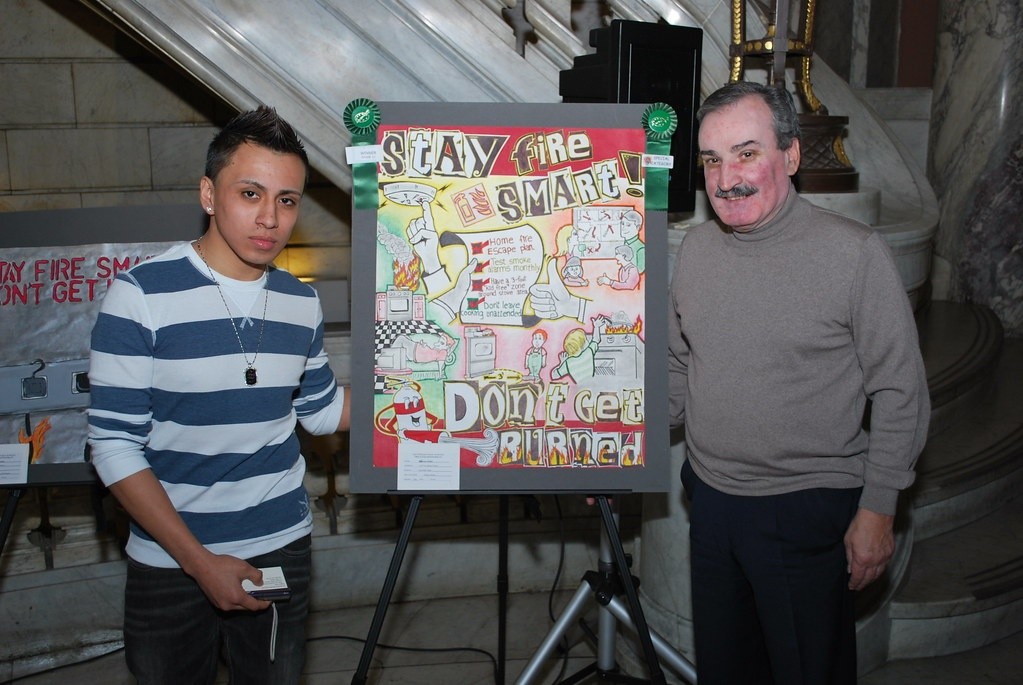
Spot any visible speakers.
[558,19,703,216]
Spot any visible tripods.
[516,494,698,685]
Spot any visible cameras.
[241,562,291,601]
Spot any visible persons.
[584,83,932,685]
[88,104,352,684]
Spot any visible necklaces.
[196,238,270,386]
